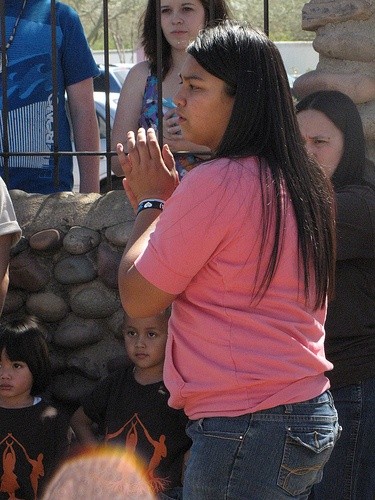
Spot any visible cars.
[63,90,124,195]
[272,40,320,89]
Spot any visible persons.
[110,0,230,182]
[0,175,22,317]
[294,90,375,500]
[0,313,80,500]
[117,21,342,500]
[42,448,162,500]
[0,0,100,194]
[70,303,193,500]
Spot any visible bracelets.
[136,199,164,215]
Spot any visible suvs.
[94,61,138,93]
